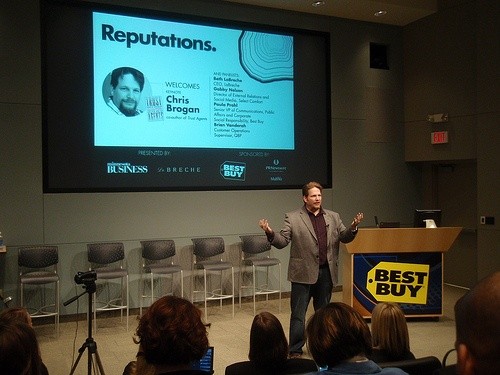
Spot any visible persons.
[369,301,415,364]
[305,302,408,375]
[106,67,145,117]
[122,295,214,375]
[0,307,49,375]
[454,272,500,375]
[261,181,364,359]
[249,311,289,364]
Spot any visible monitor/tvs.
[414,209,442,228]
[192,346,214,375]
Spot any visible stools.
[87,242,129,334]
[239,235,282,317]
[17,245,60,340]
[139,240,184,320]
[190,237,235,323]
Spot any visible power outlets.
[479,215,486,225]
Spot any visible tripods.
[62,286,105,375]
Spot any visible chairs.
[378,355,442,375]
